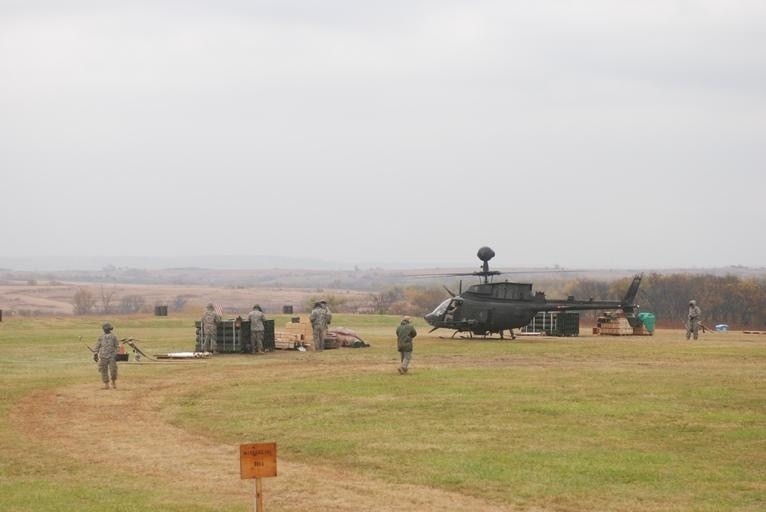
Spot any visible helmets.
[253,304,261,310]
[313,300,327,308]
[102,322,113,330]
[206,302,215,308]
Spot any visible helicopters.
[402,246,643,340]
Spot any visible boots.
[111,380,117,389]
[397,367,408,375]
[100,382,110,390]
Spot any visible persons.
[308,297,333,352]
[444,301,459,322]
[247,303,265,354]
[93,320,120,391]
[396,316,417,376]
[201,303,219,354]
[685,300,702,340]
